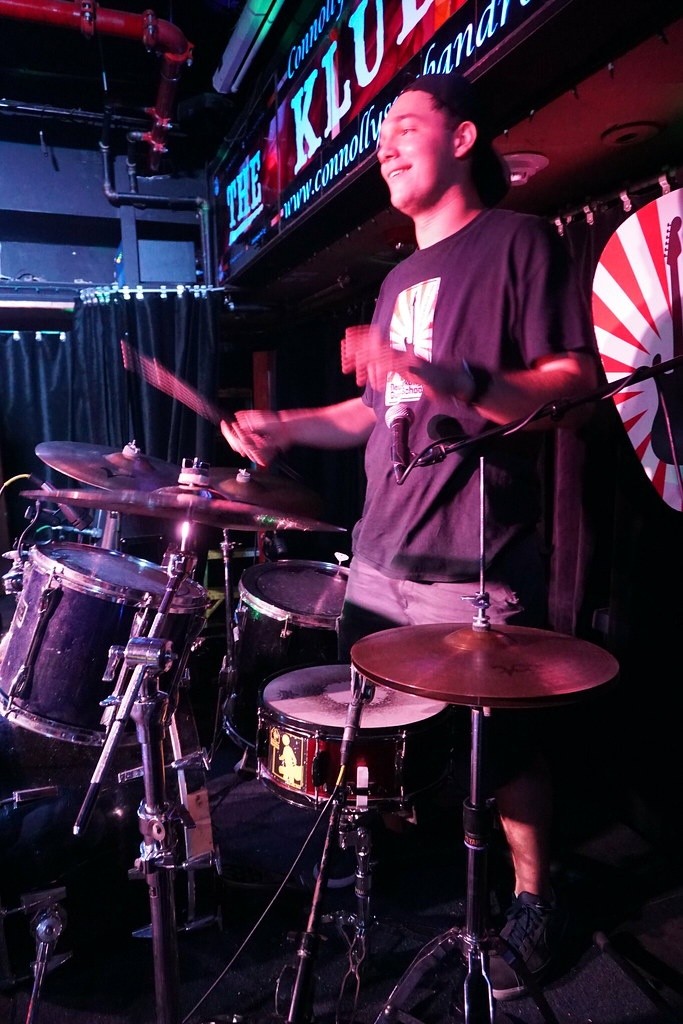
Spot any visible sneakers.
[314,823,401,886]
[487,893,566,1001]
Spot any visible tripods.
[275,706,561,1024]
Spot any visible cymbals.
[349,622,620,711]
[18,484,345,535]
[36,440,179,491]
[208,466,314,510]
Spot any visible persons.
[220,73,604,1000]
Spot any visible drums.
[0,540,211,745]
[220,559,352,755]
[255,662,456,821]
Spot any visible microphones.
[385,403,415,484]
[28,474,94,531]
[273,530,288,555]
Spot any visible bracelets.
[462,359,492,411]
